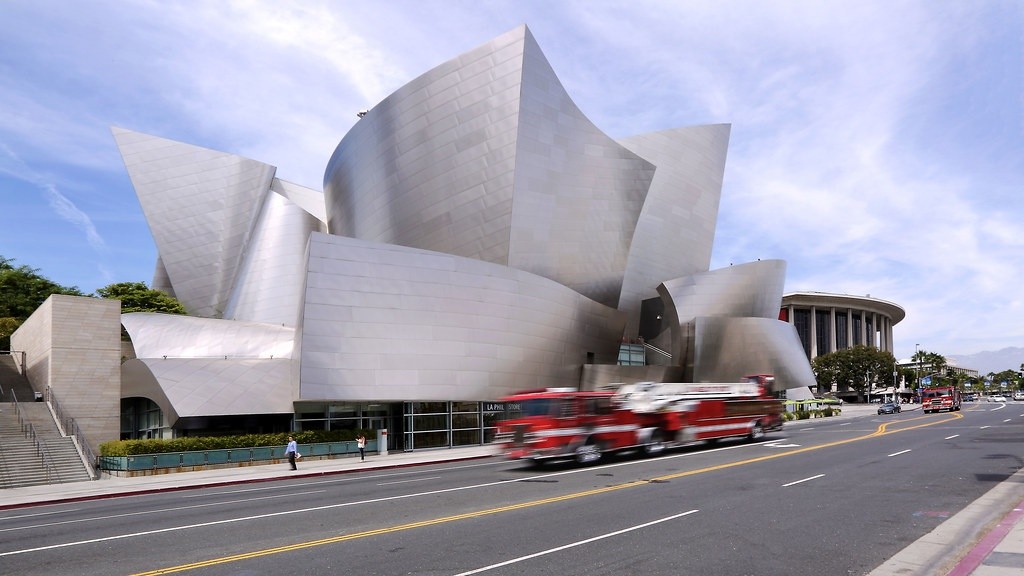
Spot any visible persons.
[914,395,918,403]
[356,431,365,463]
[284,435,298,470]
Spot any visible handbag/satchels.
[295,453,301,459]
[364,438,368,446]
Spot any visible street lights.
[915,343,920,387]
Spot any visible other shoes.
[290,468,297,471]
[359,460,364,462]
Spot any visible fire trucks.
[489,374,786,470]
[920,385,962,414]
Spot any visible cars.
[877,403,901,415]
[1014,392,1024,400]
[986,395,1008,403]
[961,394,978,402]
